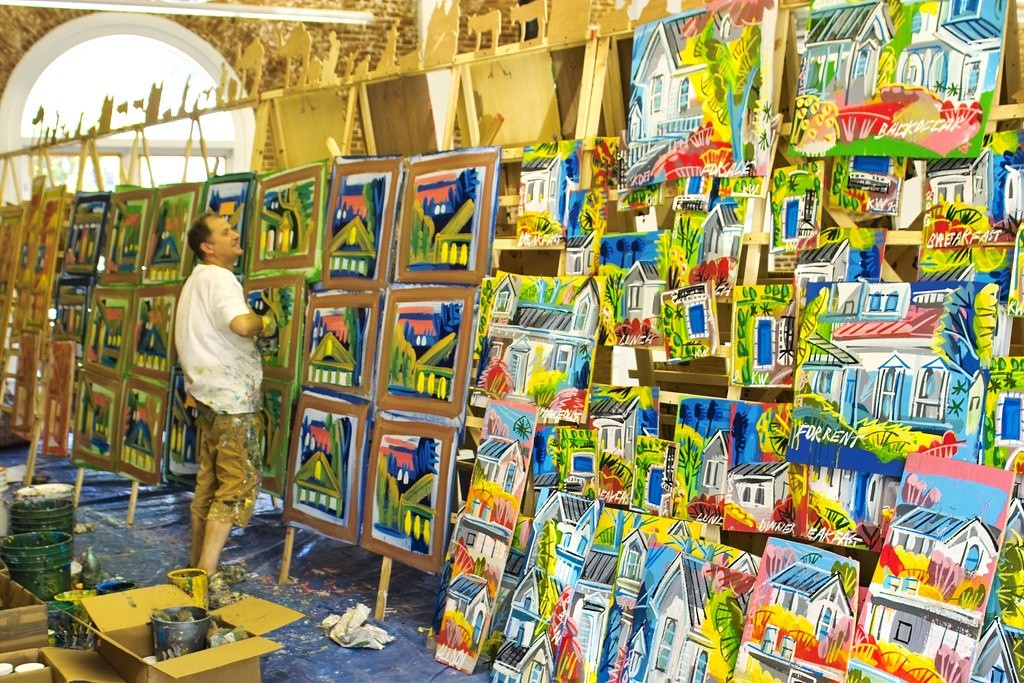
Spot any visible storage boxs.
[0,646,125,683]
[56,585,307,683]
[0,573,48,653]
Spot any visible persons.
[173,214,276,608]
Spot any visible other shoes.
[186,562,247,585]
[205,570,253,609]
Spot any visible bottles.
[81,546,100,589]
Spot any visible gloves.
[259,309,278,338]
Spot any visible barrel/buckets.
[150,606,211,662]
[0,483,136,648]
[167,568,209,613]
